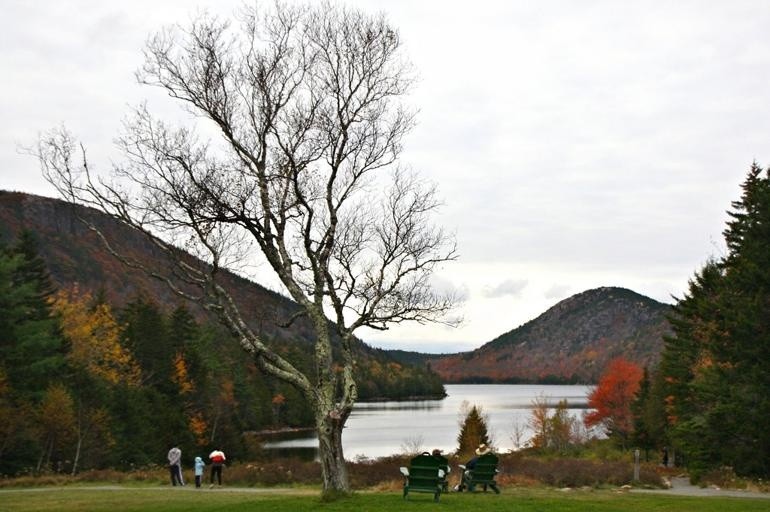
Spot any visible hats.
[475,443,491,456]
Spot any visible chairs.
[402,452,500,503]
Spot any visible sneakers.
[209,484,222,488]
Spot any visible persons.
[465,443,498,480]
[167,444,185,486]
[208,448,226,488]
[194,457,205,487]
[432,449,449,464]
